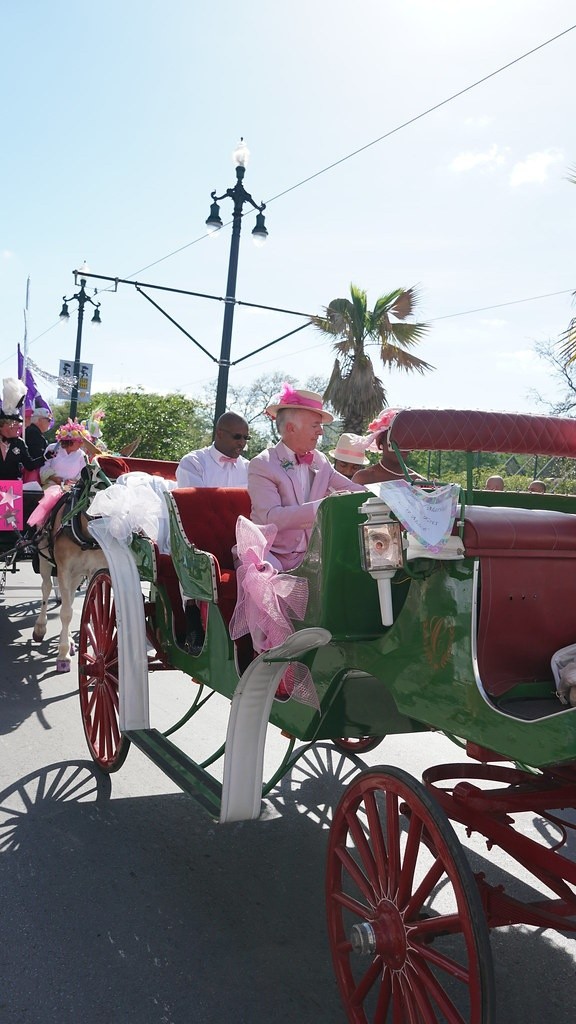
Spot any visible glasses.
[42,417,52,423]
[222,428,252,441]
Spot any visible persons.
[328,433,370,479]
[21,407,53,460]
[38,416,88,489]
[247,383,371,570]
[17,408,34,438]
[351,406,429,486]
[78,419,107,462]
[527,481,546,493]
[0,408,53,481]
[484,475,504,490]
[175,412,251,655]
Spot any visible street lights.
[59,261,102,424]
[205,137,269,443]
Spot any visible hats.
[0,378,28,423]
[56,420,90,443]
[80,401,107,439]
[368,408,412,436]
[265,387,334,424]
[329,431,370,465]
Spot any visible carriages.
[0,420,142,672]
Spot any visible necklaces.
[378,460,406,476]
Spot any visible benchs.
[103,456,178,482]
[382,406,576,688]
[167,486,253,652]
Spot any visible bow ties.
[220,455,237,463]
[295,451,314,465]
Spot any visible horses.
[33,435,142,672]
